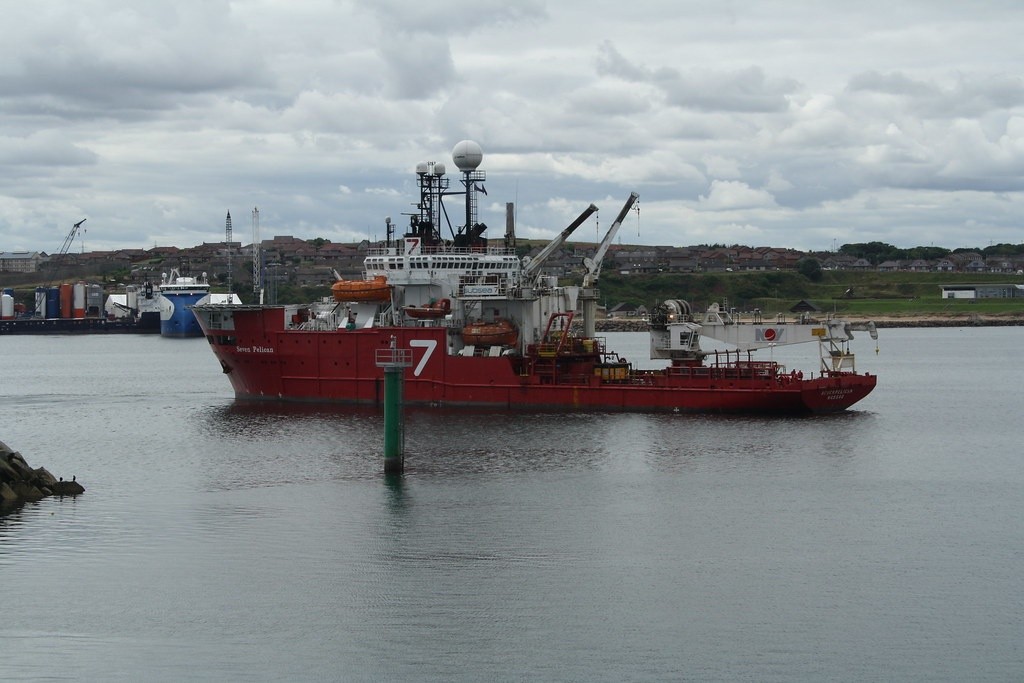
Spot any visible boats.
[401,297,454,319]
[188,139,878,419]
[329,275,393,302]
[461,320,518,348]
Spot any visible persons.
[791,369,803,379]
[642,371,654,384]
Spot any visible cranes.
[21,217,88,320]
